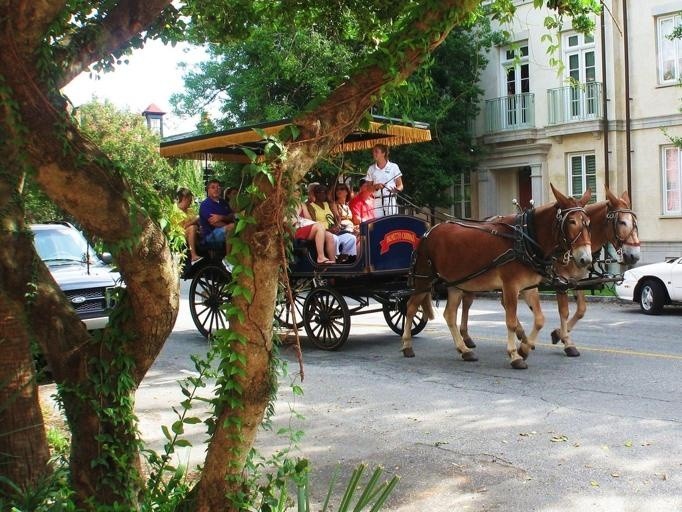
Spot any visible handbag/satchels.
[326,214,341,235]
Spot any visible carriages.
[160,110,641,368]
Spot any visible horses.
[459,183,642,361]
[400,182,593,370]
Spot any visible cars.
[615,256,682,314]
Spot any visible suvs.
[27,221,124,331]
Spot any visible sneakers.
[221,257,235,274]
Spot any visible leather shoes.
[191,256,204,266]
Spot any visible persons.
[366,144,404,219]
[172,187,201,262]
[198,178,374,264]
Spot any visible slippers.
[317,259,335,264]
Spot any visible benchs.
[196,218,317,270]
